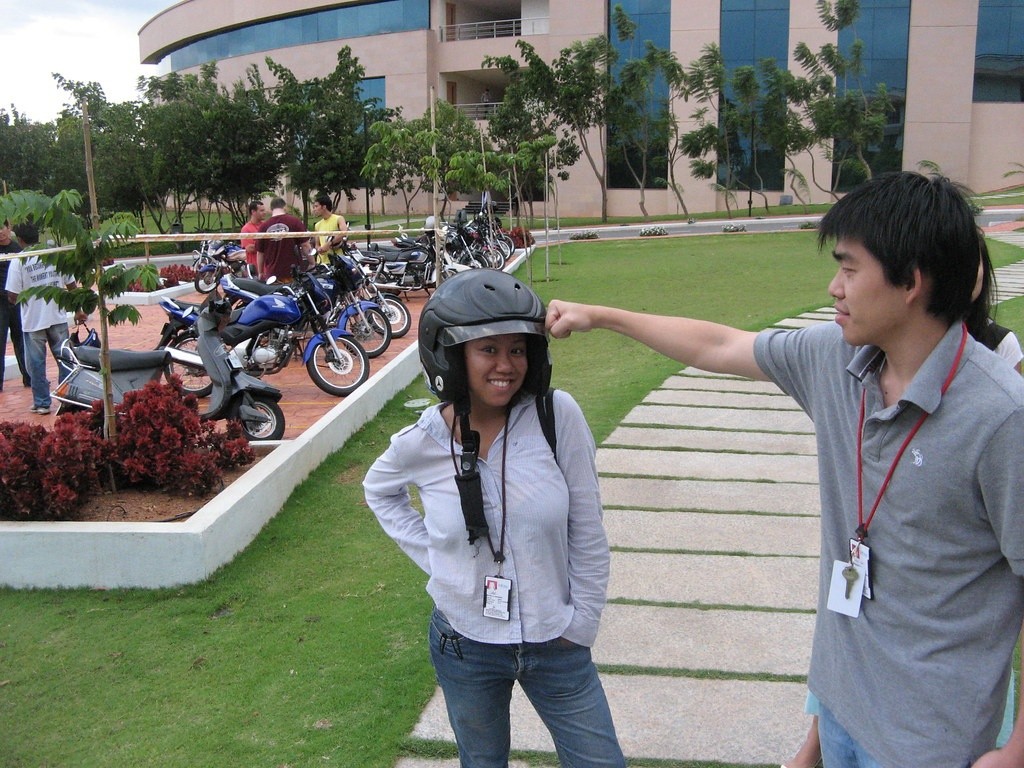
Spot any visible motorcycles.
[153,200,516,399]
[49,294,286,449]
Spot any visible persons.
[363,266,624,768]
[240,201,267,277]
[253,197,316,283]
[313,193,348,266]
[0,217,30,390]
[4,224,87,414]
[546,169,1024,768]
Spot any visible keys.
[842,567,860,599]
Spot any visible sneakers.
[28,402,51,414]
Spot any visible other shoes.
[23,378,33,387]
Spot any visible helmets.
[70,328,101,350]
[418,268,553,401]
